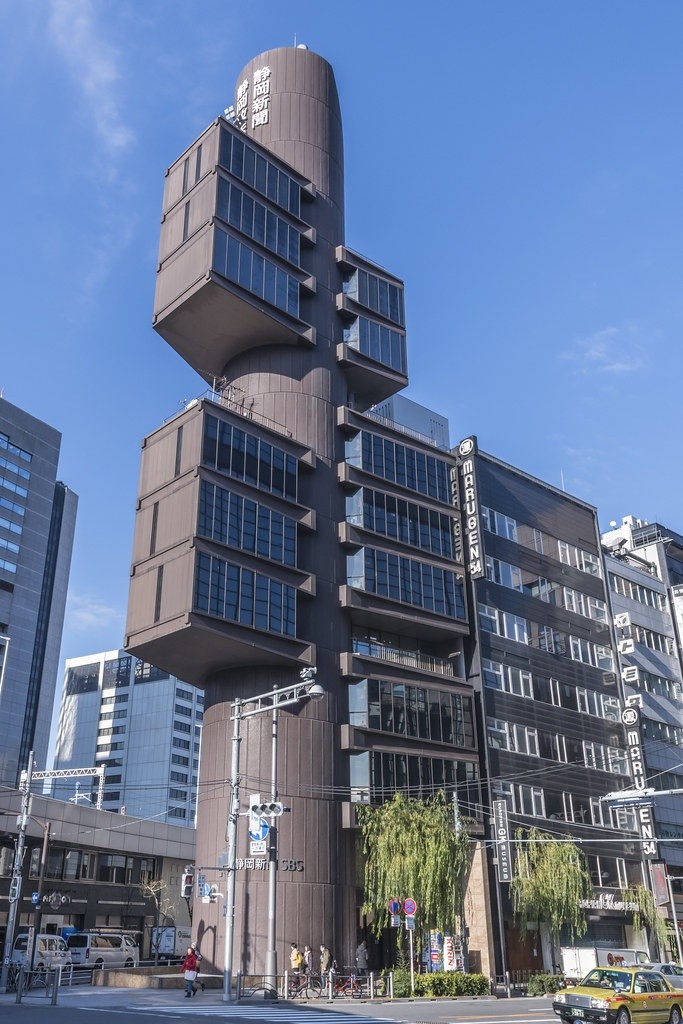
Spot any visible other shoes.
[185,990,188,992]
[185,993,191,998]
[192,989,197,996]
[201,983,205,991]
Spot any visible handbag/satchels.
[185,970,197,980]
[196,961,200,967]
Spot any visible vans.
[68,933,140,970]
[11,933,73,973]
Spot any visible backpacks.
[295,951,302,964]
[329,953,333,967]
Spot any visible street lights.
[15,812,52,990]
[224,679,326,1000]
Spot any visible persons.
[601,975,615,988]
[180,942,205,997]
[303,944,313,988]
[319,944,334,989]
[289,943,303,988]
[354,939,370,985]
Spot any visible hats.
[191,942,197,948]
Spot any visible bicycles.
[326,966,363,1000]
[282,967,322,999]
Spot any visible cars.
[550,965,683,1024]
[633,962,683,989]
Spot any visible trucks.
[560,945,652,985]
